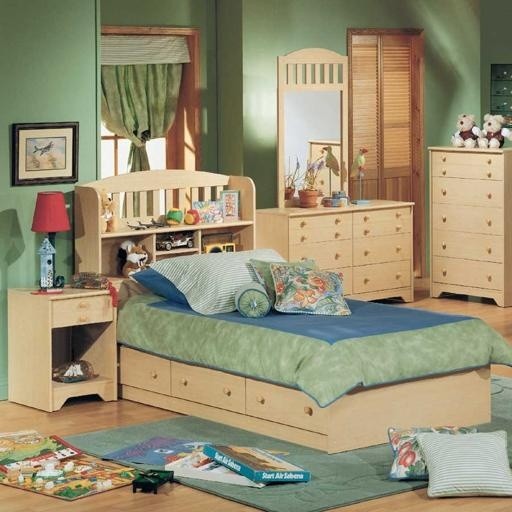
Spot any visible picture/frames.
[9,115,83,188]
[219,189,241,220]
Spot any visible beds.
[70,165,511,454]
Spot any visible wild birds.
[322,146,340,177]
[349,147,368,177]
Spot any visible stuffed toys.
[100,190,117,234]
[450,113,481,147]
[117,239,148,278]
[477,114,512,149]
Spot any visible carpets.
[51,372,511,510]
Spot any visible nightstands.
[5,282,120,415]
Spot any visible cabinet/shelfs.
[305,138,344,197]
[250,198,418,305]
[424,143,511,311]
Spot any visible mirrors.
[270,44,355,206]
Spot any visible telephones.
[71,272,109,290]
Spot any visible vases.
[297,187,326,208]
[283,184,297,200]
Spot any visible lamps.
[28,190,72,292]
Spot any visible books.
[165,450,269,488]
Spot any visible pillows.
[264,261,353,318]
[248,255,318,307]
[410,427,512,499]
[125,265,188,307]
[385,424,478,484]
[233,280,272,320]
[146,243,287,316]
